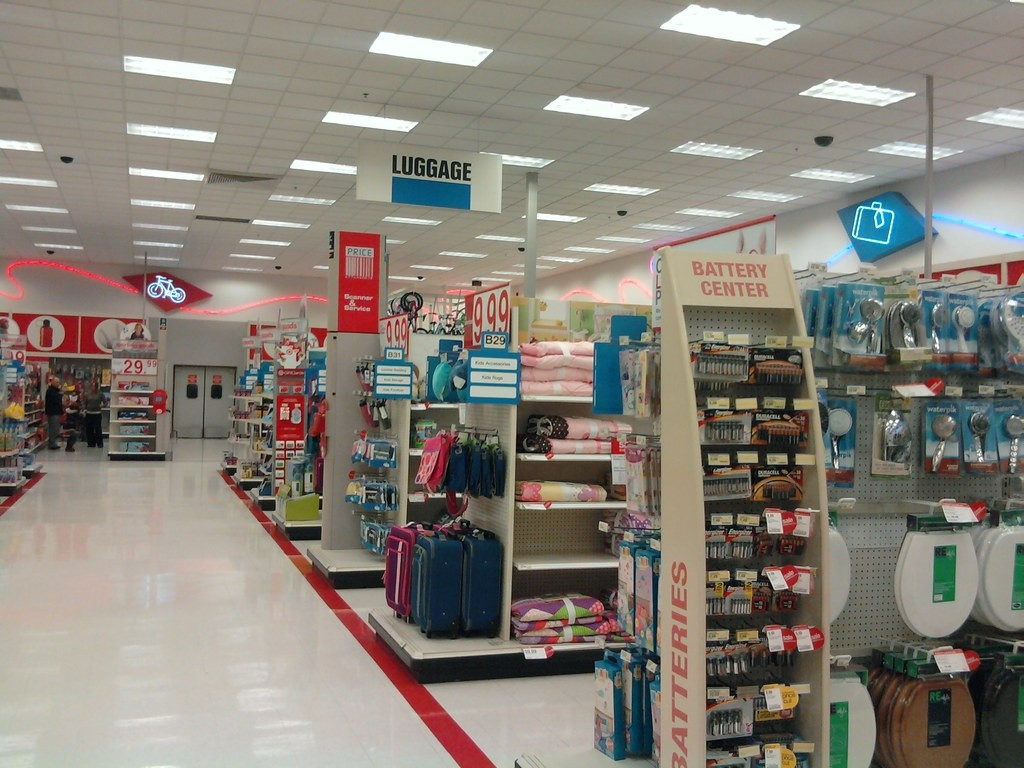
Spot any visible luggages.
[386,517,504,639]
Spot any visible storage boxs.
[118,411,148,420]
[118,395,149,406]
[118,425,149,436]
[117,380,150,391]
[118,441,148,452]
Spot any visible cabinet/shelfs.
[306,308,466,588]
[220,292,327,540]
[0,359,45,496]
[370,295,658,683]
[591,314,660,767]
[107,339,166,461]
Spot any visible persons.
[130,323,145,340]
[45,375,108,452]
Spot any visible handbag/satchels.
[414,431,506,498]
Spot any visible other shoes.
[98,444,104,448]
[50,446,61,450]
[66,448,75,452]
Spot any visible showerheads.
[818,297,1023,474]
